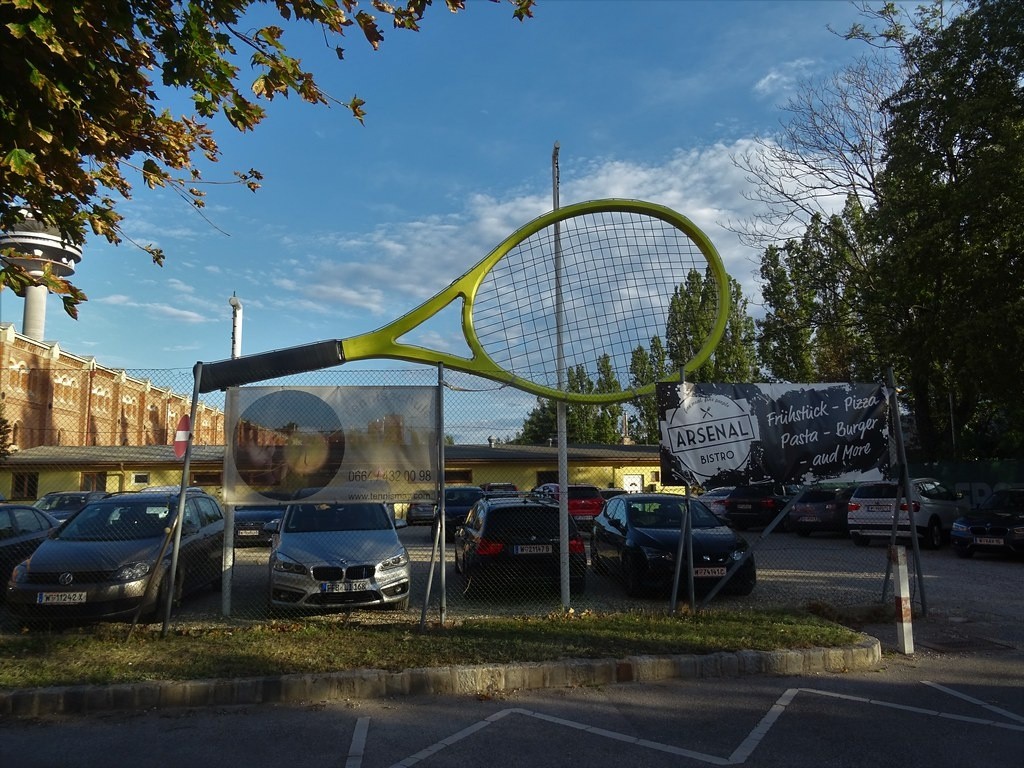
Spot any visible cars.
[591,492,756,596]
[699,487,735,522]
[432,487,483,543]
[533,483,606,532]
[950,489,1023,559]
[479,482,517,497]
[599,489,627,499]
[268,503,412,619]
[406,489,438,526]
[234,492,286,548]
[0,506,62,599]
[361,478,396,527]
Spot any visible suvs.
[8,485,235,633]
[455,497,588,602]
[789,487,846,536]
[846,477,955,549]
[32,490,111,523]
[725,481,795,529]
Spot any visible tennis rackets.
[193,196,732,407]
